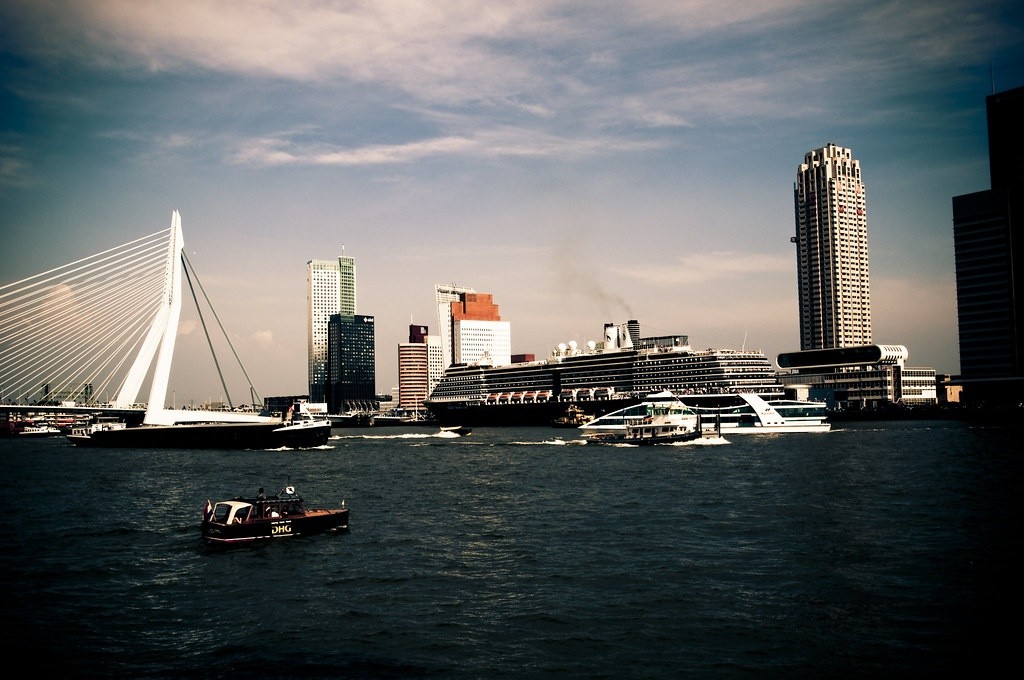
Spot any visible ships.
[423,319,786,427]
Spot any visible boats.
[19,422,61,436]
[554,404,595,427]
[440,426,473,436]
[66,418,332,449]
[200,476,350,547]
[588,401,702,445]
[577,388,831,435]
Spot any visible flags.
[207,503,212,513]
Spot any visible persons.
[256,487,266,499]
[272,508,279,517]
[233,517,242,524]
